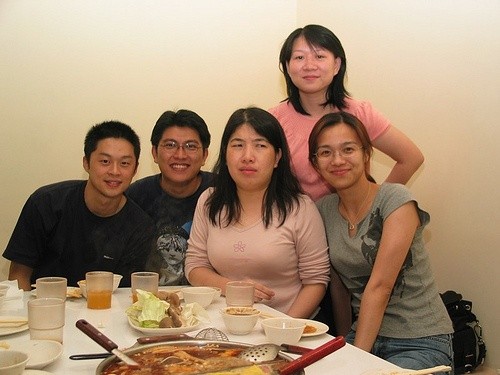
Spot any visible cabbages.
[126,288,208,327]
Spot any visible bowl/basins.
[219,307,261,335]
[112,274,123,291]
[0,349,30,375]
[77,279,88,299]
[260,317,306,344]
[182,287,215,309]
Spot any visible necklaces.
[340,182,371,229]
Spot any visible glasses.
[313,145,364,160]
[156,141,204,153]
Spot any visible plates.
[0,312,30,337]
[0,339,63,369]
[159,286,192,300]
[298,320,329,337]
[23,368,52,375]
[29,286,83,299]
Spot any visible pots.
[94,340,306,375]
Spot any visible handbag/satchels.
[435,290,487,375]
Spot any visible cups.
[85,271,115,310]
[27,297,65,342]
[130,271,159,305]
[36,276,67,303]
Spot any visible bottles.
[224,281,255,309]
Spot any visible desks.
[0,286,402,375]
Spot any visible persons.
[3,120,148,293]
[125,108,220,287]
[308,111,455,375]
[185,107,331,321]
[261,25,425,203]
